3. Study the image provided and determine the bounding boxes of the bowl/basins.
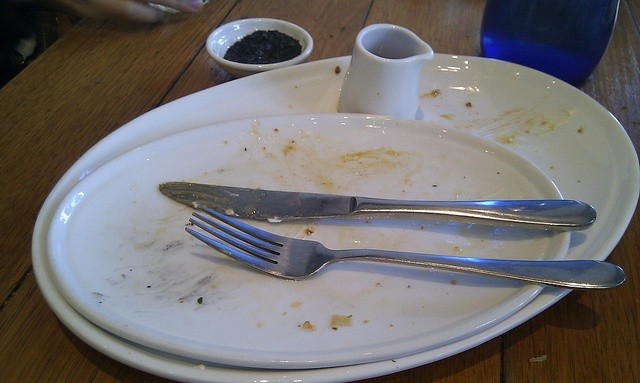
[207,17,313,76]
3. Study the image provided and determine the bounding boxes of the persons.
[1,1,205,86]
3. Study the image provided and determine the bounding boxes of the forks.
[183,205,625,293]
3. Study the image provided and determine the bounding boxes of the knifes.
[157,182,596,231]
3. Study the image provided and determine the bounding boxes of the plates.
[48,111,572,370]
[32,52,640,382]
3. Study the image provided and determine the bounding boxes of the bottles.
[337,22,433,119]
[481,1,618,88]
